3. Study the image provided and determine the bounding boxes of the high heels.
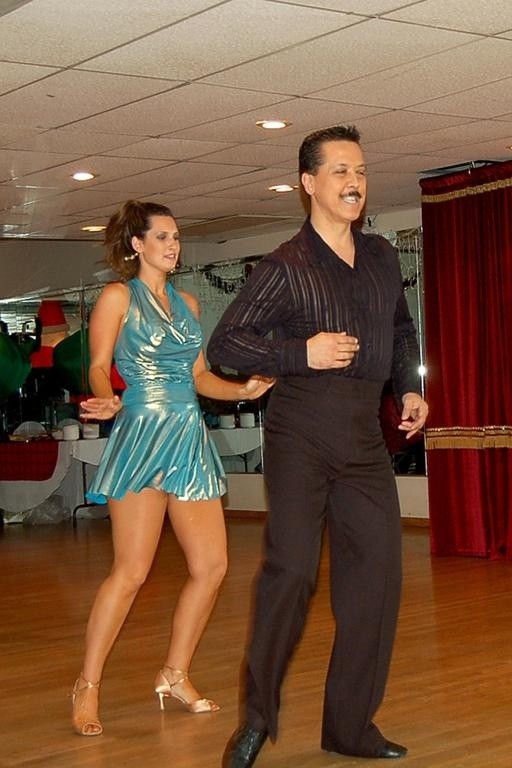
[152,666,222,715]
[71,671,102,737]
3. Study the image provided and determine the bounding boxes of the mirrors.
[0,227,429,476]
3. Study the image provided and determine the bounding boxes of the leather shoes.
[222,722,268,767]
[377,739,407,758]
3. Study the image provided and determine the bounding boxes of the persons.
[68,197,278,738]
[203,124,432,766]
[376,376,427,478]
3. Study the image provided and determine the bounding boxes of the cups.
[218,412,256,429]
[62,423,99,442]
